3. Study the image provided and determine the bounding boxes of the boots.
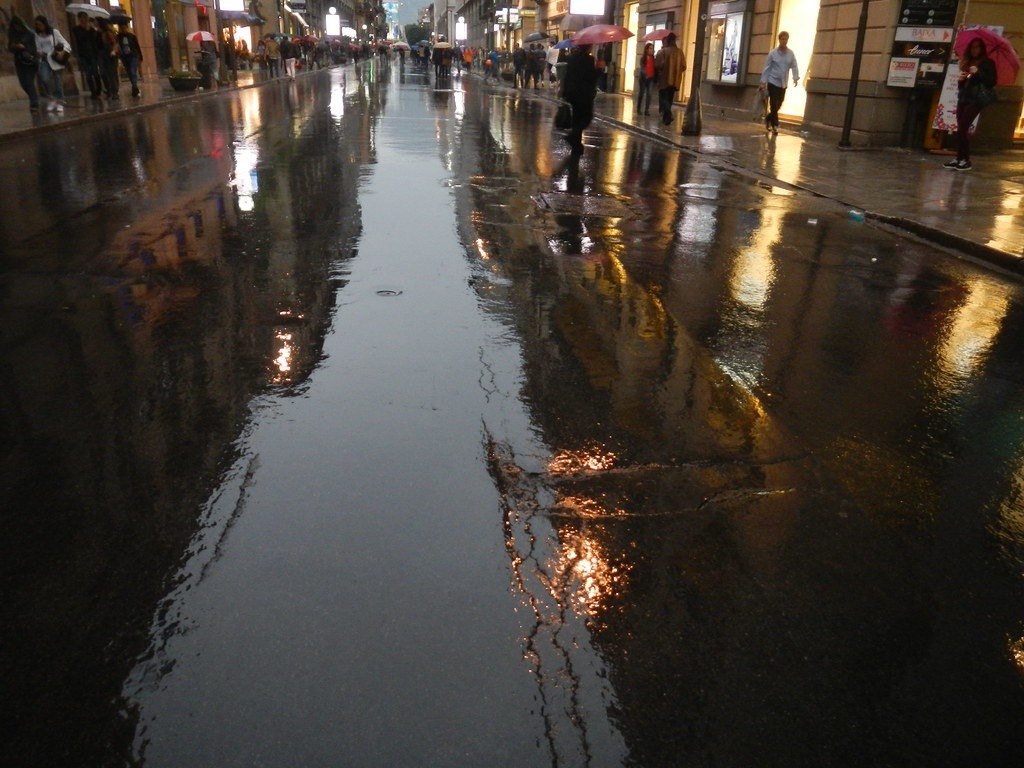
[637,91,644,114]
[645,95,651,115]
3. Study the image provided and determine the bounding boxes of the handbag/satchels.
[555,106,571,129]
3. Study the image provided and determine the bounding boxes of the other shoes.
[764,117,770,128]
[289,78,291,82]
[942,161,959,168]
[772,128,778,133]
[292,79,296,81]
[954,162,972,171]
[541,83,544,87]
[566,134,583,154]
[534,87,539,90]
[512,86,516,88]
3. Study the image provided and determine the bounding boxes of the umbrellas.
[106,13,133,24]
[570,24,636,44]
[65,3,110,20]
[263,31,452,50]
[554,39,577,49]
[642,28,681,40]
[524,31,550,43]
[954,28,1021,86]
[186,30,217,41]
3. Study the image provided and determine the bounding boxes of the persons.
[760,31,800,135]
[194,40,219,92]
[637,32,687,126]
[942,36,998,170]
[237,34,608,94]
[557,44,605,166]
[7,12,143,112]
